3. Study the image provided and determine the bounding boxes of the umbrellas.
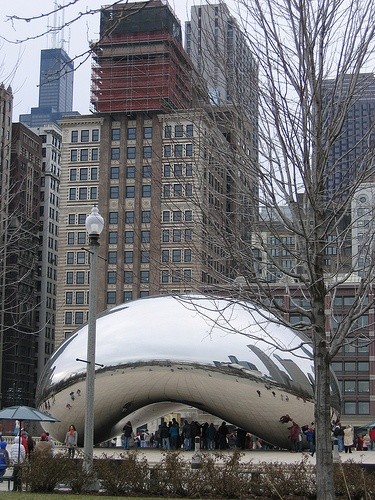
[0,405,62,464]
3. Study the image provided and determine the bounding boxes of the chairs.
[0,463,18,491]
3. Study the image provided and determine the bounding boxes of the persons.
[333,423,355,453]
[0,442,10,483]
[200,421,229,450]
[123,421,132,449]
[28,435,36,453]
[11,435,25,465]
[64,425,78,460]
[297,423,316,456]
[40,431,53,441]
[182,420,201,451]
[356,426,375,451]
[21,431,30,459]
[236,427,274,450]
[14,420,23,437]
[136,428,163,448]
[160,418,179,450]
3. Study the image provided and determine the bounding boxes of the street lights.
[77,201,106,493]
